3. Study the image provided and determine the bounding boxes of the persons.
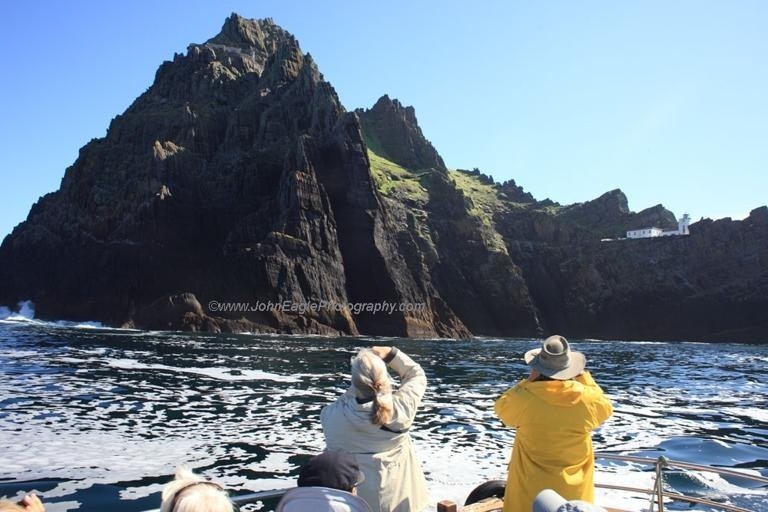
[273,450,375,512]
[319,347,428,511]
[533,489,609,511]
[0,495,45,512]
[491,336,613,511]
[160,470,234,512]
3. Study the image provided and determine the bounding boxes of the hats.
[296,450,366,490]
[523,333,588,383]
[530,488,607,512]
